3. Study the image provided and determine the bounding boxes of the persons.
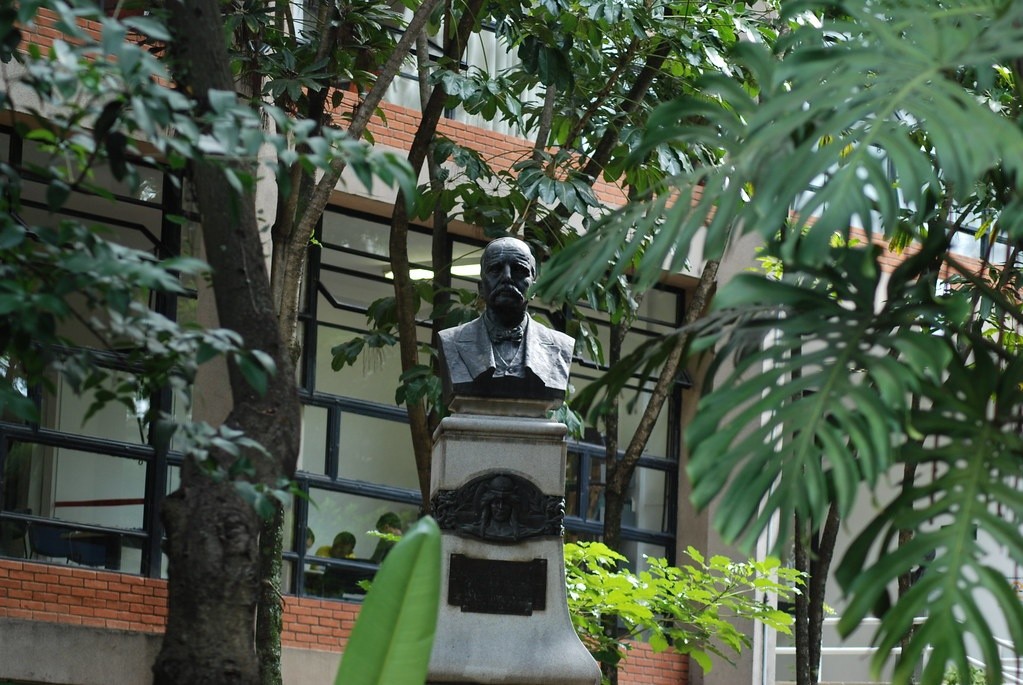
[376,511,402,560]
[310,532,356,570]
[306,527,315,549]
[438,236,575,400]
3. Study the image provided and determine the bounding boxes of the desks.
[62,530,167,570]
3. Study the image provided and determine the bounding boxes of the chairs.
[4,507,74,562]
